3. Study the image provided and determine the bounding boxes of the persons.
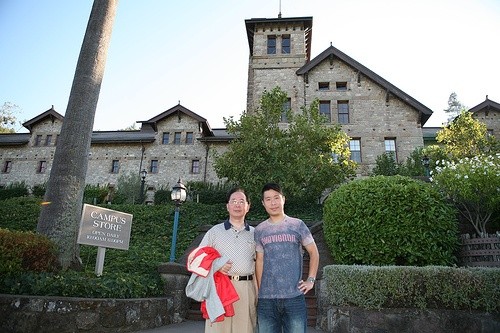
[253,185,320,333]
[196,188,258,333]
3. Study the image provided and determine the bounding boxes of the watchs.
[306,276,316,284]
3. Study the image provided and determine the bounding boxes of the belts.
[227,275,253,280]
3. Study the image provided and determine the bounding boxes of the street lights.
[139,169,147,204]
[168,177,188,261]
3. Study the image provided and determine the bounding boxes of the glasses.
[229,200,247,205]
